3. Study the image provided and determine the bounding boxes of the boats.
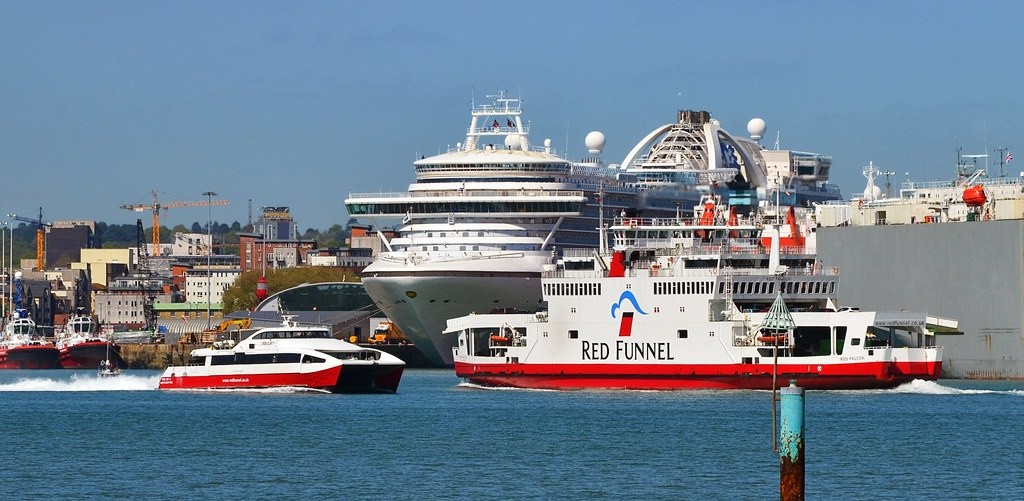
[159,326,409,399]
[0,311,63,370]
[439,182,966,393]
[96,361,123,379]
[55,307,127,369]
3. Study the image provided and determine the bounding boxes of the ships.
[345,87,992,369]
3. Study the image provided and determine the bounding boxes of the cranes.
[120,187,233,257]
[134,217,158,332]
[6,204,52,274]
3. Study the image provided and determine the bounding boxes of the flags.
[1005,152,1013,164]
